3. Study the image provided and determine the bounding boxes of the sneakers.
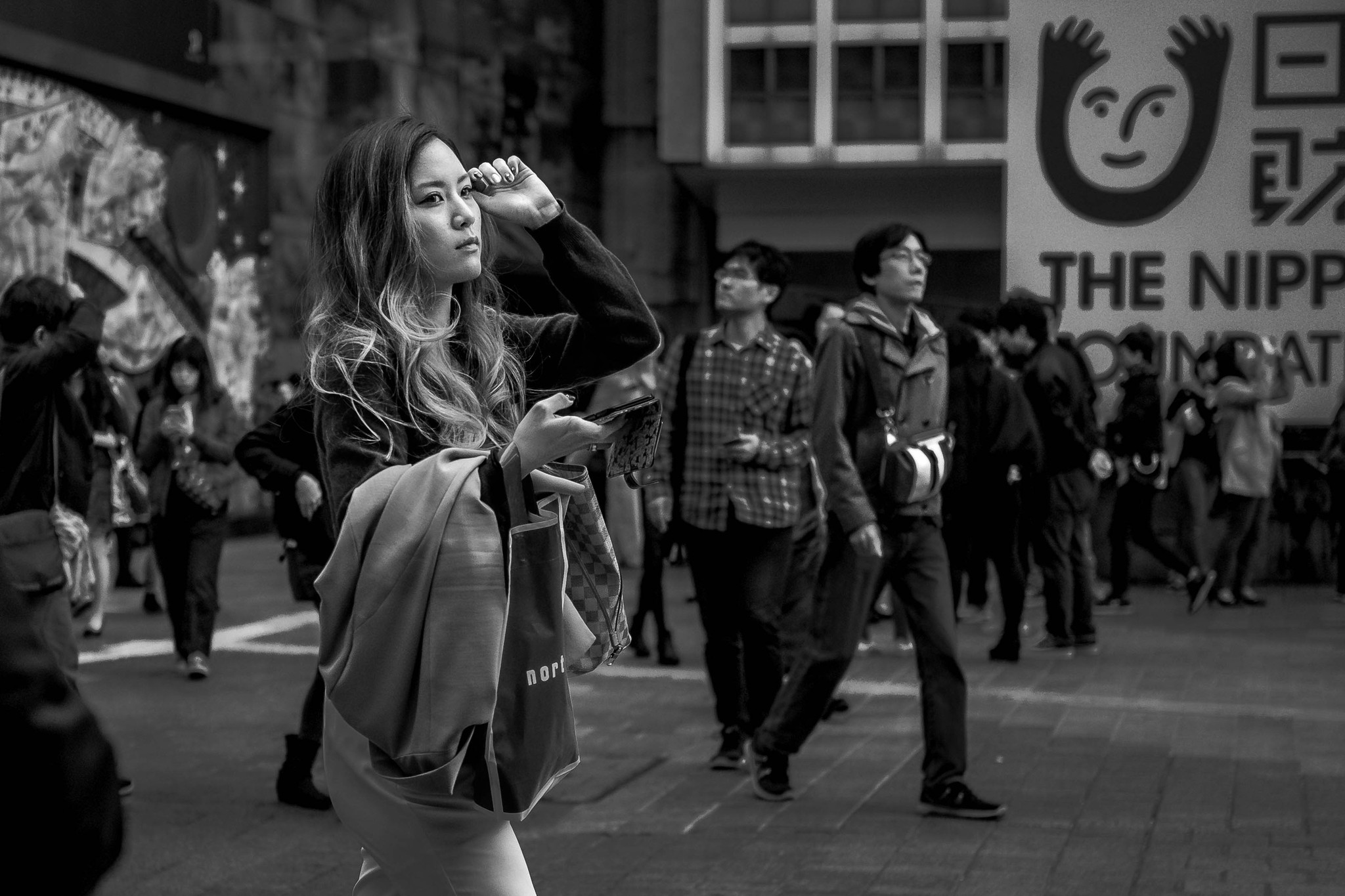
[1093,592,1133,611]
[916,781,1007,819]
[1186,568,1217,615]
[709,741,748,769]
[175,654,209,682]
[744,739,795,801]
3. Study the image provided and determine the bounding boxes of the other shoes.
[858,641,876,652]
[1207,585,1267,609]
[120,776,135,796]
[896,641,914,652]
[1031,630,1097,648]
[143,588,164,615]
[81,613,103,637]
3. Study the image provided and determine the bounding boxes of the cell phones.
[583,394,655,423]
[167,408,187,425]
[722,438,742,446]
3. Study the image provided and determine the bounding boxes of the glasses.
[712,266,761,283]
[880,246,934,266]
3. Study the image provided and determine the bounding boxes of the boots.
[629,613,649,658]
[114,534,146,589]
[657,628,676,665]
[989,615,1019,661]
[276,733,334,811]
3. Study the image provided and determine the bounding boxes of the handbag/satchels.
[879,428,956,505]
[495,401,634,671]
[659,487,690,567]
[290,551,335,604]
[0,508,80,673]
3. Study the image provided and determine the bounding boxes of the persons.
[573,284,1298,663]
[742,221,1009,819]
[0,273,166,896]
[307,120,666,896]
[135,331,239,680]
[651,242,817,770]
[232,392,336,814]
